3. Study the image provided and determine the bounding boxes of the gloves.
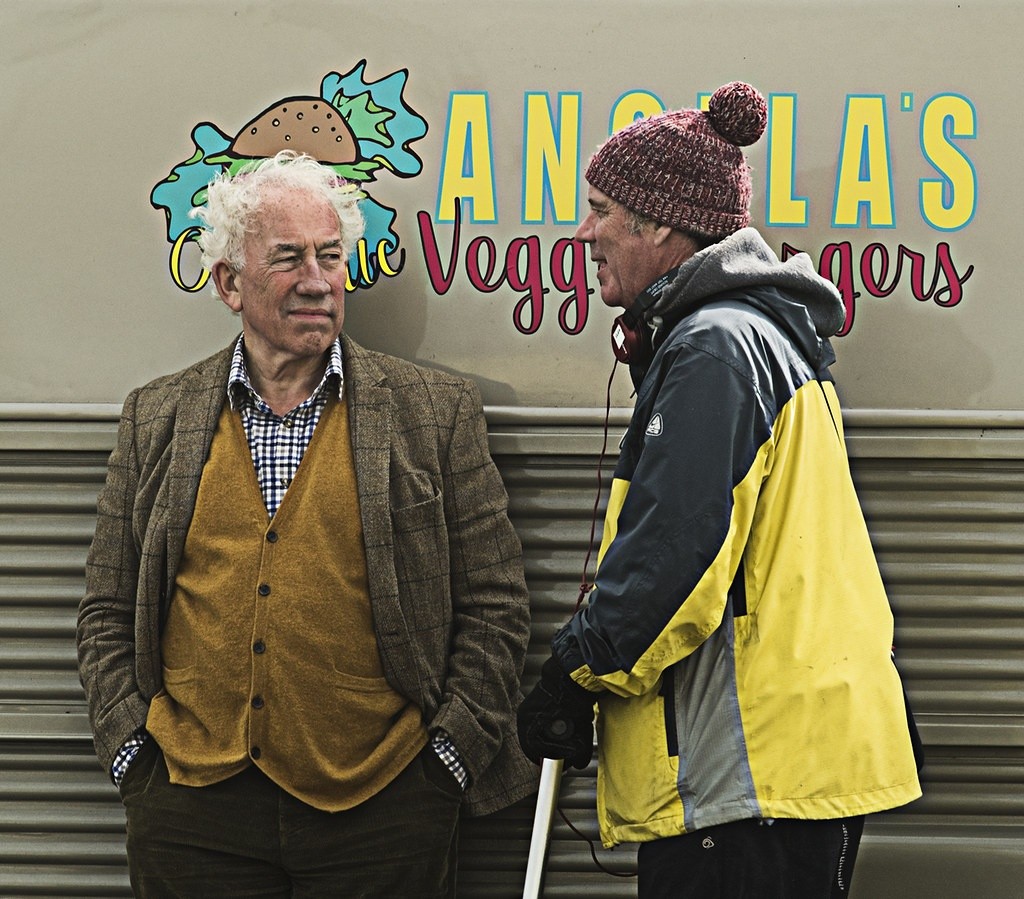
[515,658,595,770]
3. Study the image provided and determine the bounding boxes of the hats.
[587,80,766,239]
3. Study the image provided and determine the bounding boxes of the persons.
[75,149,533,899]
[517,80,925,899]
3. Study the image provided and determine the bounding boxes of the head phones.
[610,266,681,363]
